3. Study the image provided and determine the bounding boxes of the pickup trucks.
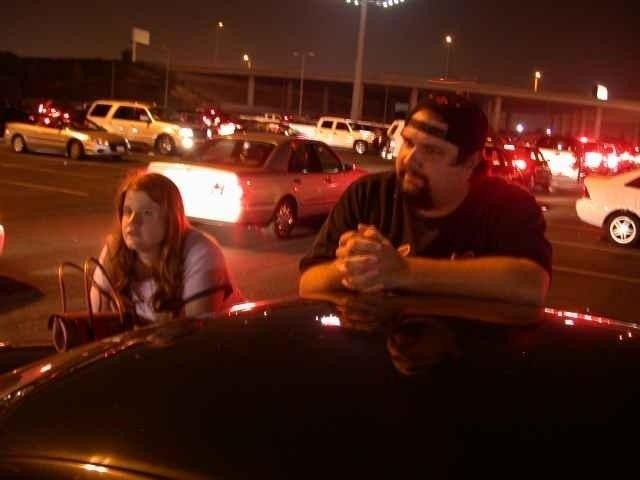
[288,116,377,155]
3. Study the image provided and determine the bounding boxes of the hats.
[404,89,489,150]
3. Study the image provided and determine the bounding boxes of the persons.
[89,173,248,329]
[307,291,548,377]
[298,94,552,309]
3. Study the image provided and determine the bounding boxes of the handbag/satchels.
[48,257,129,353]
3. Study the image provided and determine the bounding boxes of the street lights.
[344,0,407,122]
[444,35,452,80]
[291,50,313,116]
[213,20,225,63]
[534,71,541,94]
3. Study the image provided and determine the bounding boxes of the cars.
[240,111,285,132]
[380,120,406,159]
[0,286,639,477]
[579,137,640,175]
[148,133,377,242]
[531,134,583,182]
[483,144,553,194]
[576,167,640,249]
[4,98,131,162]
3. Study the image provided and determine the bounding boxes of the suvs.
[85,99,195,156]
